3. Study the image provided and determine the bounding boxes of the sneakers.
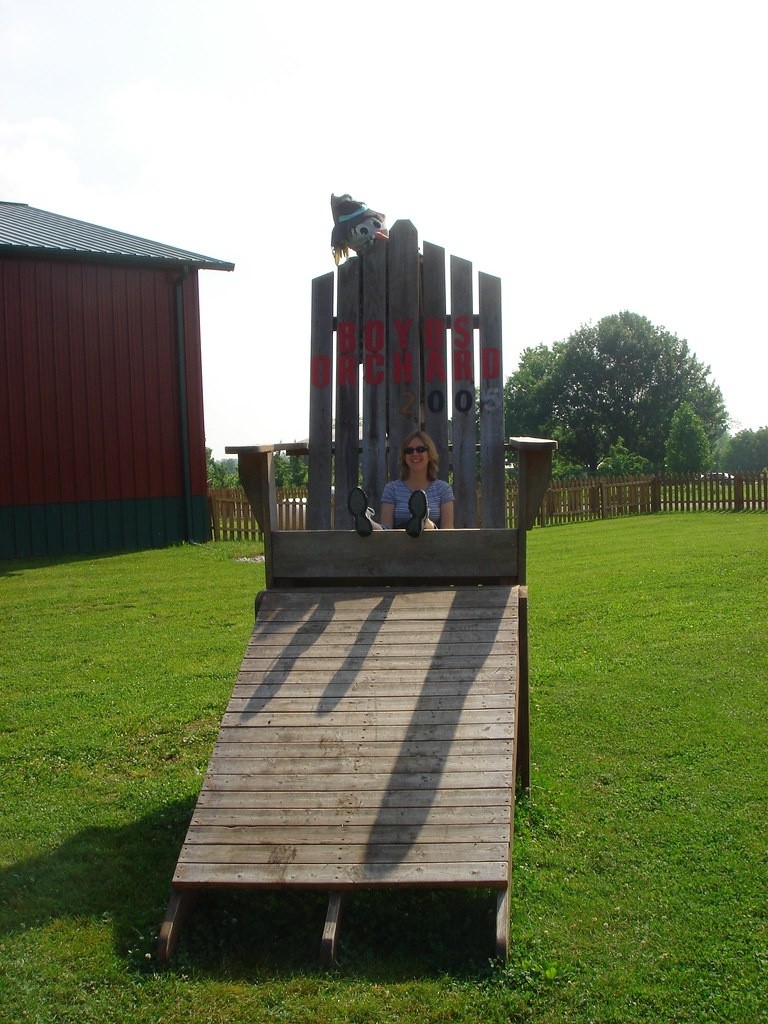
[406,490,429,538]
[348,486,372,537]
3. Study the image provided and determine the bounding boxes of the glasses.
[405,446,428,454]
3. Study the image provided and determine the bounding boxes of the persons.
[346,429,457,538]
